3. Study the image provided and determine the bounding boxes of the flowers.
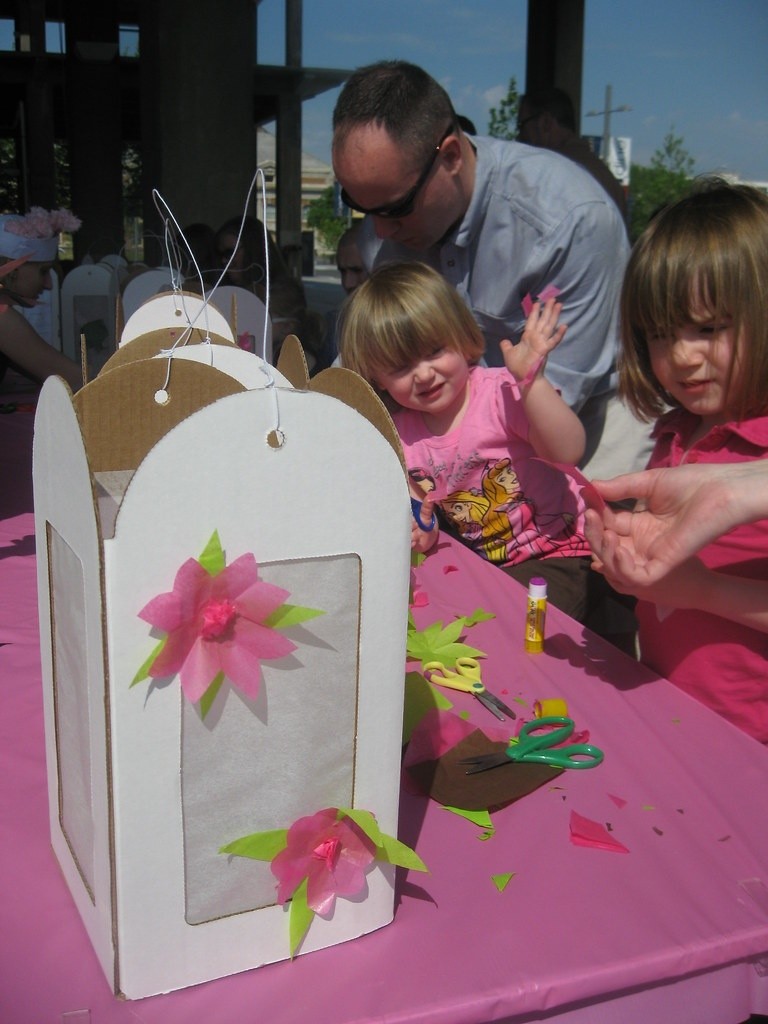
[127,528,326,723]
[218,806,428,962]
[6,206,81,239]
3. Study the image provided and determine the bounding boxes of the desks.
[0,512,768,1024]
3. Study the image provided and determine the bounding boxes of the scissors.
[457,716,604,775]
[423,657,516,721]
[411,496,435,531]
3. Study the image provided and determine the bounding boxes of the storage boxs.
[17,254,413,1001]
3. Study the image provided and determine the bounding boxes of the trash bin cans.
[302,229,314,276]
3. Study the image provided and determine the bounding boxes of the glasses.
[340,125,456,219]
[517,112,541,129]
[214,244,246,261]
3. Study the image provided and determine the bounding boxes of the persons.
[324,221,375,369]
[332,61,630,473]
[584,184,768,747]
[0,207,92,394]
[335,258,610,626]
[161,215,327,378]
[515,85,628,222]
